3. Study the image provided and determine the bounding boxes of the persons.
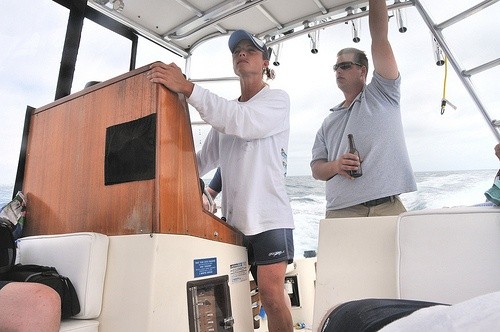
[147,28,295,332]
[310,0,418,219]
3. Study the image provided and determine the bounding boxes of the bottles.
[347,134,362,177]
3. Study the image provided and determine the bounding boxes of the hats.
[228,29,268,56]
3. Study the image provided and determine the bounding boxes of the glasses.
[333,61,362,71]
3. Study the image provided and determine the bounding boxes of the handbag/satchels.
[0,262,80,319]
[0,190,27,241]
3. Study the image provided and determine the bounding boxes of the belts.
[361,194,397,207]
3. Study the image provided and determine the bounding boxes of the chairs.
[397,207,500,304]
[14,232,109,332]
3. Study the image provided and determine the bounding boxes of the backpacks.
[0,222,17,266]
